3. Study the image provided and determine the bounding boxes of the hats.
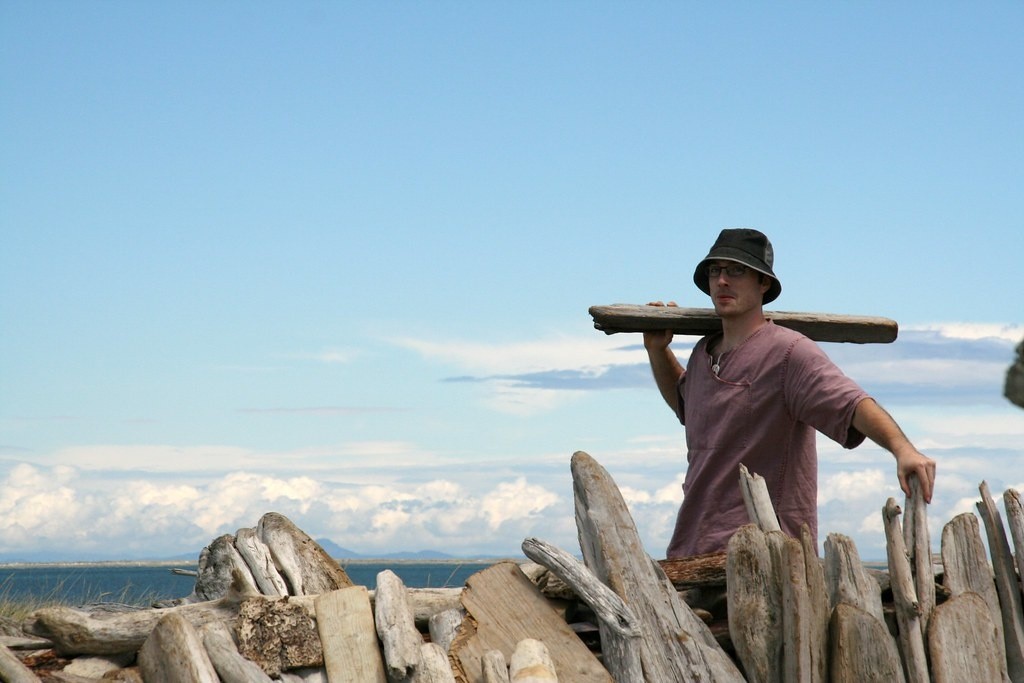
[693,228,782,305]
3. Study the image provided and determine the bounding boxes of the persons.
[643,229,935,559]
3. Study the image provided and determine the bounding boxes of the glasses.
[709,264,754,277]
